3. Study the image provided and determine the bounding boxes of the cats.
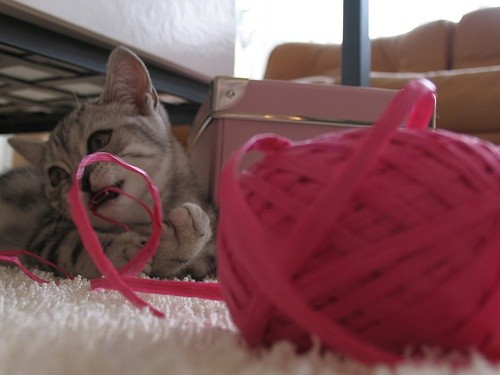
[0,44,217,282]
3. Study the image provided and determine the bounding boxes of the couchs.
[266,7,500,145]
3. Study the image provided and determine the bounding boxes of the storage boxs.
[184,74,438,212]
